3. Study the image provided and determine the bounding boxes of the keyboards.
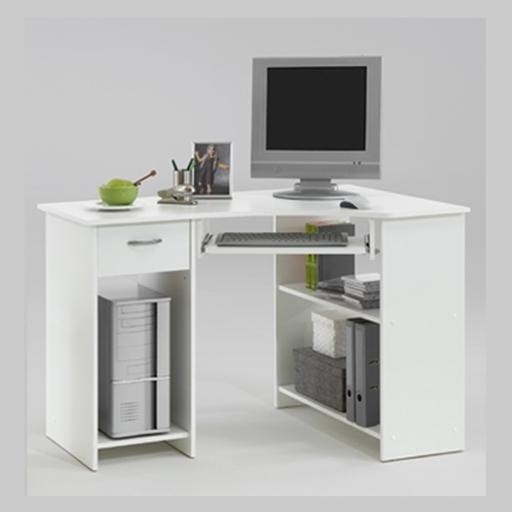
[216,231,349,247]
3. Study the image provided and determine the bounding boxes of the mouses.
[339,193,369,210]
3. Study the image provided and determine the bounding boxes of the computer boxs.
[98,280,172,439]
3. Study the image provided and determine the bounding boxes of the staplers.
[157,185,198,205]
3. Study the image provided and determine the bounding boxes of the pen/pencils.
[172,157,195,170]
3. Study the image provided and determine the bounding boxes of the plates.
[93,200,141,210]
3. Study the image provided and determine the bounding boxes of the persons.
[195,145,229,194]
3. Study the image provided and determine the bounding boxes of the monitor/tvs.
[250,56,383,200]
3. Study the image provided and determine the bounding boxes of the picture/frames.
[189,139,234,200]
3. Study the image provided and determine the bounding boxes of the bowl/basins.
[98,186,139,206]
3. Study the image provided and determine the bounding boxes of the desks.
[37,182,472,471]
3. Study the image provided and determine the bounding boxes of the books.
[316,272,380,310]
